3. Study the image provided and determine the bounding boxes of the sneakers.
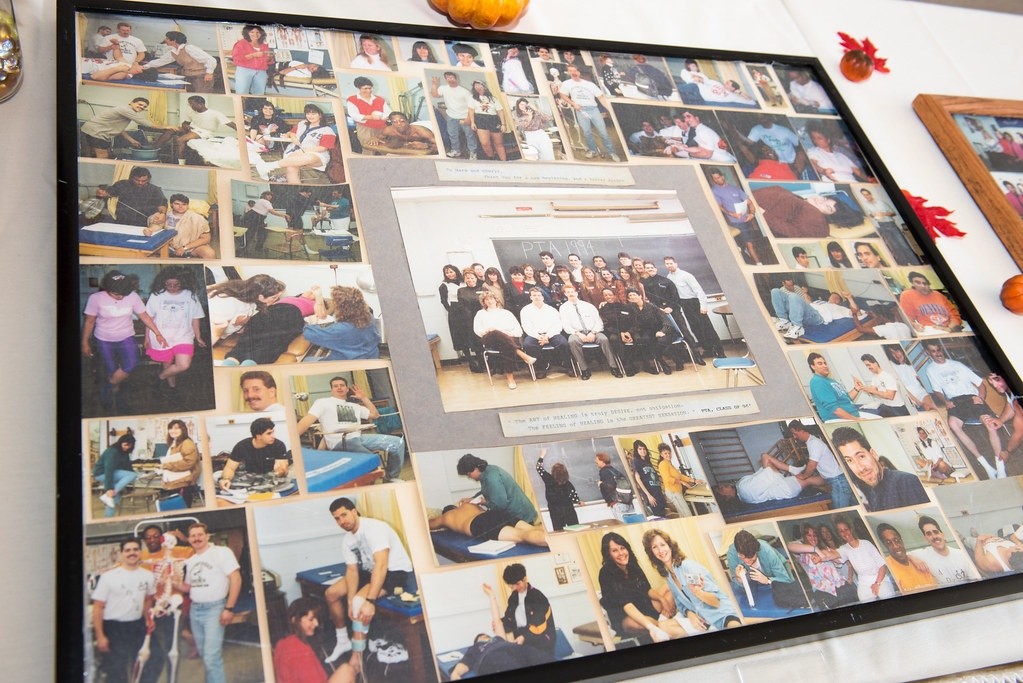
[776,318,792,331]
[368,639,409,664]
[784,325,805,338]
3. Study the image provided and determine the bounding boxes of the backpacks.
[608,468,633,504]
[635,65,659,97]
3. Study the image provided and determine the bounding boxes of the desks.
[78,222,178,259]
[712,304,735,345]
[296,562,428,683]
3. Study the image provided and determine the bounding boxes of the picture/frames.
[912,93,1023,272]
[54,0,1023,683]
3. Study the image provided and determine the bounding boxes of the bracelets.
[854,387,859,392]
[182,246,187,250]
[365,599,376,602]
[876,582,880,585]
[294,67,297,70]
[157,335,162,336]
[768,579,771,585]
[814,546,815,552]
[224,607,234,612]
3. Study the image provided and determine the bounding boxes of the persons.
[82,23,1023,683]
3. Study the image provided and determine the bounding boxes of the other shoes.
[537,362,550,379]
[525,356,537,364]
[447,149,461,157]
[696,355,707,366]
[99,493,115,508]
[586,150,598,158]
[582,368,590,380]
[718,350,725,359]
[611,152,620,162]
[508,379,516,389]
[470,151,477,160]
[676,359,683,371]
[661,361,671,376]
[701,351,713,358]
[610,366,623,378]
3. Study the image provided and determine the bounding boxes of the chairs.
[712,340,766,389]
[267,230,358,263]
[481,312,703,384]
